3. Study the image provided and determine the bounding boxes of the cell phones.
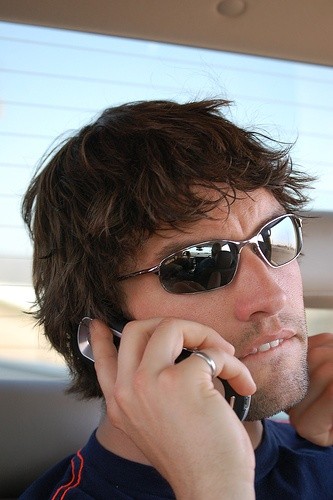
[75,316,251,422]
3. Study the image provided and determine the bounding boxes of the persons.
[19,98,332,500]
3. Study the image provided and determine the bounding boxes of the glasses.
[115,213,304,295]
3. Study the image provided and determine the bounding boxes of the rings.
[193,350,215,381]
